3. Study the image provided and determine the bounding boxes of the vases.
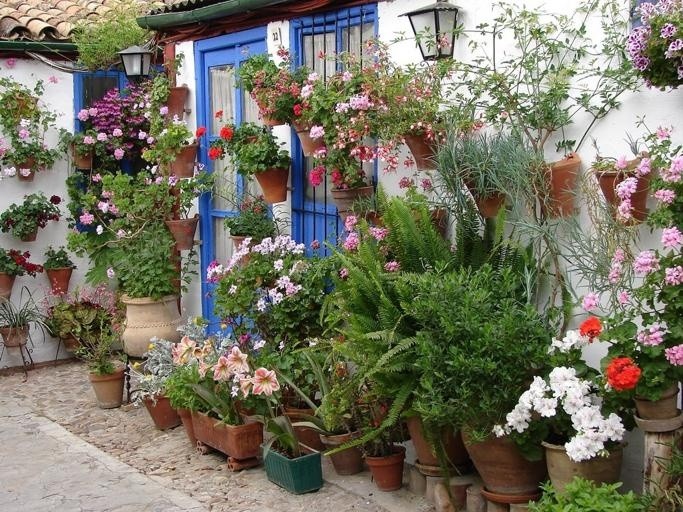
[366,446,406,490]
[460,425,546,495]
[541,439,622,499]
[264,440,322,495]
[320,434,366,476]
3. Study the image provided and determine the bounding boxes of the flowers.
[0,0,683,460]
